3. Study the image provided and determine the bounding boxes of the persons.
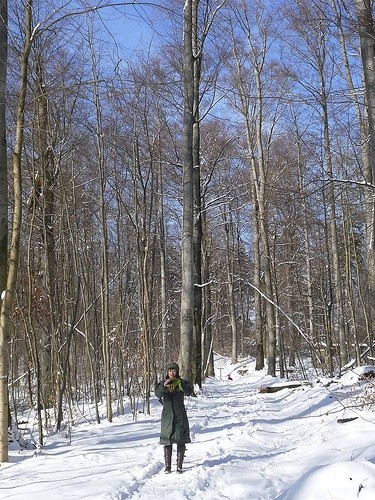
[155,362,194,474]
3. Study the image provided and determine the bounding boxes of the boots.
[163,445,171,474]
[176,445,186,473]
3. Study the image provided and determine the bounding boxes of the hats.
[166,363,179,375]
